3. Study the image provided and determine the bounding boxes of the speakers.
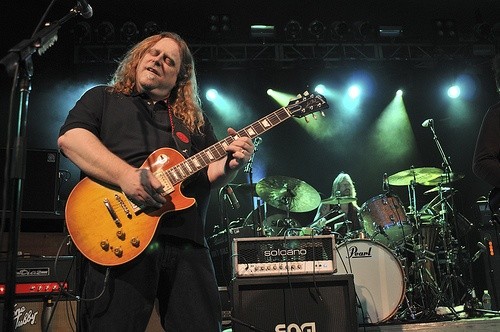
[0,147,60,213]
[0,295,79,332]
[228,274,357,332]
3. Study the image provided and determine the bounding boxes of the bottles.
[482,290,491,309]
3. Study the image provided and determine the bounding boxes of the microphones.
[76,0,93,19]
[227,184,241,209]
[421,118,433,127]
[245,137,262,173]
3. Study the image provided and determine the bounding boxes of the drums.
[412,219,442,297]
[335,238,407,325]
[359,192,414,246]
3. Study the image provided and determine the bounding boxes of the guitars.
[64,89,331,268]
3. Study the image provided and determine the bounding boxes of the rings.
[241,149,245,153]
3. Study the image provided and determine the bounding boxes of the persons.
[313,174,365,238]
[56,32,255,332]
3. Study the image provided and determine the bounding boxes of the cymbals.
[422,186,455,199]
[385,166,444,185]
[262,214,302,237]
[255,175,322,213]
[418,172,465,186]
[320,195,358,206]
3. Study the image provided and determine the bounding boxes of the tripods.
[384,128,477,322]
